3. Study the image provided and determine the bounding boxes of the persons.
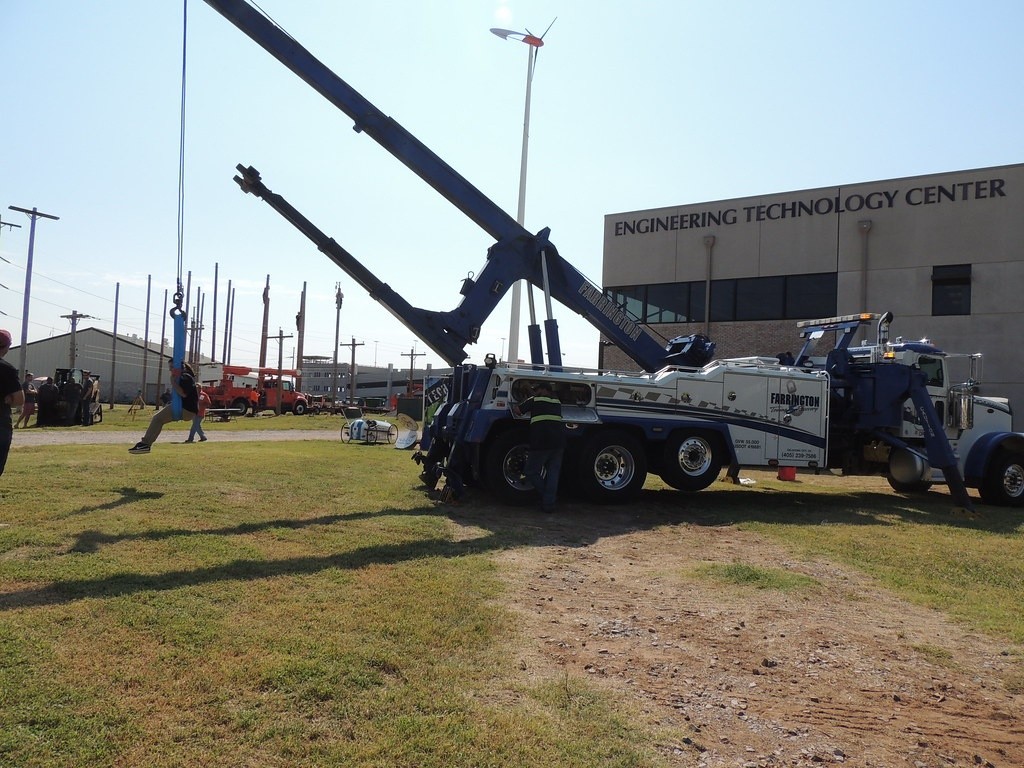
[160,388,171,408]
[36,377,59,427]
[257,389,266,410]
[63,372,94,427]
[185,383,211,442]
[251,387,258,414]
[128,358,199,454]
[514,381,566,514]
[14,373,38,428]
[0,330,26,529]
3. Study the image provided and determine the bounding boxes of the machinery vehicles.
[199,365,308,416]
[37,368,102,426]
[203,0,1024,509]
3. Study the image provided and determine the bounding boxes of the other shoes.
[185,440,193,443]
[198,437,208,442]
[128,442,151,453]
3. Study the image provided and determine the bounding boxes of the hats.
[195,383,202,388]
[0,329,12,346]
